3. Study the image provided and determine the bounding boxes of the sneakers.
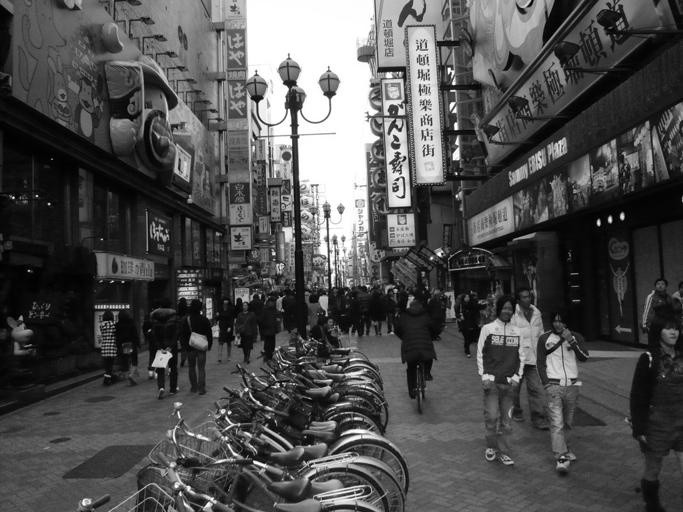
[532,416,550,430]
[425,374,433,380]
[411,392,416,399]
[566,450,576,461]
[466,352,471,358]
[512,406,523,421]
[191,388,197,393]
[170,386,179,395]
[199,390,206,394]
[485,447,496,461]
[157,387,165,399]
[148,371,154,379]
[555,455,570,473]
[498,453,514,466]
[154,372,159,379]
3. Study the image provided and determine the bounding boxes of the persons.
[509,287,548,429]
[536,308,590,474]
[94,289,297,398]
[641,276,671,333]
[671,282,682,306]
[304,286,488,400]
[475,294,526,465]
[629,310,681,512]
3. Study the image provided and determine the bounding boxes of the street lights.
[308,197,348,295]
[247,53,340,341]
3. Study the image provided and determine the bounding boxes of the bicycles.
[411,356,430,414]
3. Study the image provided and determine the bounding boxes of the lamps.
[470,9,682,146]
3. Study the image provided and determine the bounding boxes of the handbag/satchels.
[238,324,246,333]
[189,331,209,351]
[234,336,241,345]
[457,312,464,322]
[151,350,174,369]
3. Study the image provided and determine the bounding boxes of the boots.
[111,375,121,383]
[104,374,112,385]
[641,478,668,512]
[123,371,133,386]
[129,366,138,384]
